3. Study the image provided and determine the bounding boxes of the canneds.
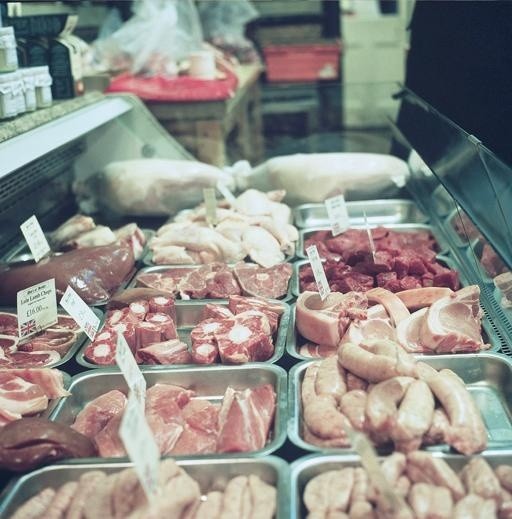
[0,25,54,119]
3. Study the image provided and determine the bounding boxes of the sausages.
[7,459,278,519]
[303,453,511,519]
[302,337,488,455]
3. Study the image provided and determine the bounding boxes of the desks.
[105,58,266,172]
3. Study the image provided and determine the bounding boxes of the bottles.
[0,26,54,121]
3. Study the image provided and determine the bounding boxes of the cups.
[187,52,218,80]
